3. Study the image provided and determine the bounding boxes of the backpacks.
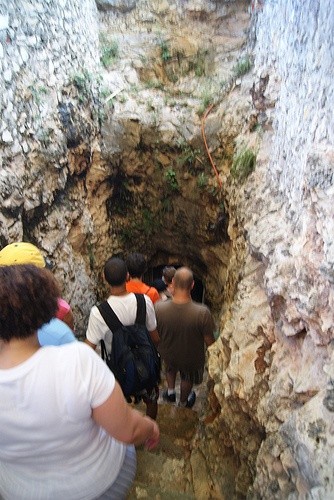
[95,293,162,399]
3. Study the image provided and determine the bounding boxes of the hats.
[0,242,46,269]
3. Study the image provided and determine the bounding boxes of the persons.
[124,256,161,307]
[0,241,79,346]
[53,298,76,333]
[85,257,162,422]
[153,280,169,302]
[160,266,178,301]
[0,262,161,500]
[153,266,217,410]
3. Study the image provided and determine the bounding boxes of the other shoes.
[178,391,196,409]
[163,388,177,403]
